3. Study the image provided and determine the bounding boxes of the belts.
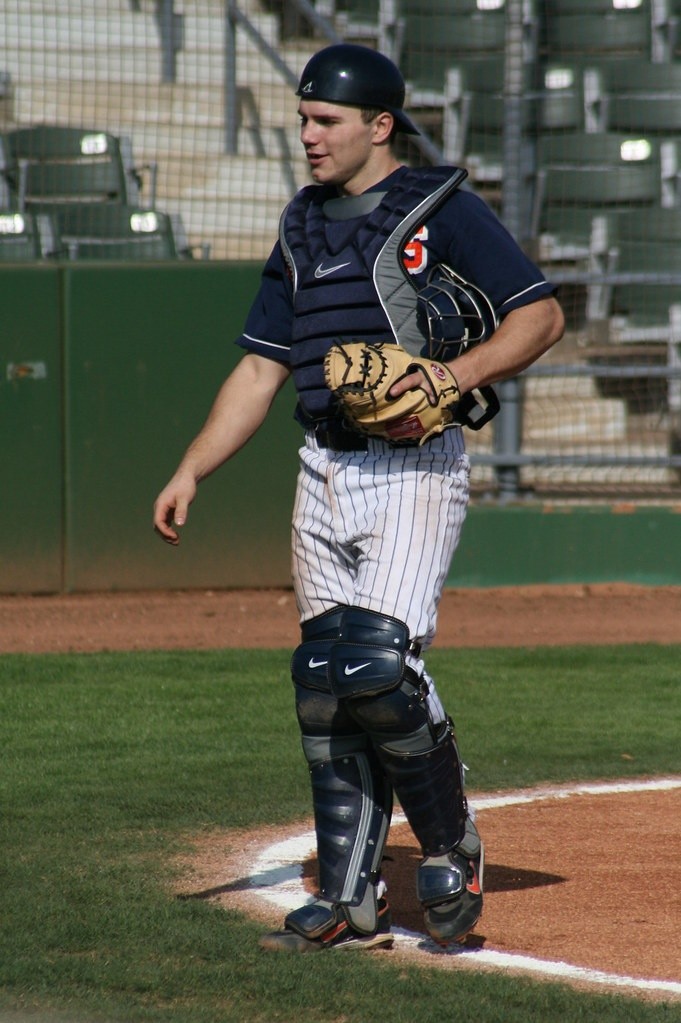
[315,429,444,453]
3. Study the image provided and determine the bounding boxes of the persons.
[153,48,566,956]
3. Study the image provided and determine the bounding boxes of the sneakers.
[257,896,394,953]
[417,816,484,949]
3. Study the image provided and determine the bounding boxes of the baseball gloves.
[323,336,465,451]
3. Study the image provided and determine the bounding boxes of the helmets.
[295,44,422,137]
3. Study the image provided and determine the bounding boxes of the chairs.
[0,0,681,411]
